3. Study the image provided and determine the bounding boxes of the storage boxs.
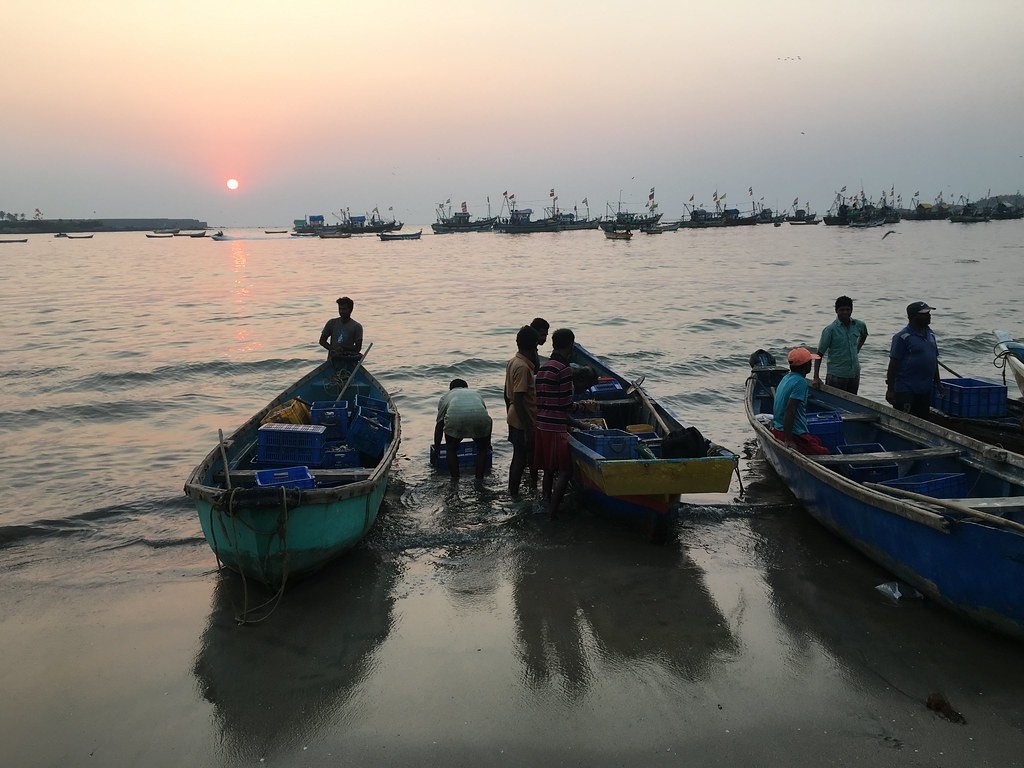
[429,440,493,471]
[250,392,394,487]
[808,377,1009,500]
[570,378,663,460]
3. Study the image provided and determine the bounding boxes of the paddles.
[333,342,373,408]
[627,375,670,435]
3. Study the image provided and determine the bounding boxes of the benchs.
[810,448,967,465]
[842,411,876,422]
[229,469,373,480]
[572,398,640,408]
[940,495,1024,512]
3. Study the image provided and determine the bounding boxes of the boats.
[604,230,632,239]
[183,349,401,582]
[265,230,287,233]
[0,240,28,243]
[153,230,180,233]
[376,230,423,239]
[567,340,740,512]
[54,232,67,237]
[319,234,351,237]
[291,210,405,233]
[68,235,95,238]
[174,232,206,236]
[291,233,317,236]
[431,192,1024,234]
[992,329,1024,396]
[146,234,173,238]
[929,397,1024,456]
[746,350,1023,634]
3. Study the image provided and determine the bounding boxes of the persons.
[319,297,363,359]
[433,379,493,480]
[885,302,945,420]
[504,318,585,506]
[812,296,868,396]
[772,348,829,456]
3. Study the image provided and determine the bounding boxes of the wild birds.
[882,230,903,240]
[777,56,802,60]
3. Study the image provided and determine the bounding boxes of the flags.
[793,197,798,205]
[503,191,507,195]
[713,192,726,201]
[461,202,467,212]
[749,187,752,196]
[582,198,587,203]
[509,194,514,199]
[861,188,900,202]
[550,189,554,196]
[649,187,654,200]
[915,191,919,196]
[841,186,846,192]
[373,208,378,212]
[689,196,694,201]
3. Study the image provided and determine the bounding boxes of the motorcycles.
[211,233,225,240]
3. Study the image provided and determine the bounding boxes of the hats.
[907,301,936,316]
[788,348,822,366]
[337,298,353,307]
